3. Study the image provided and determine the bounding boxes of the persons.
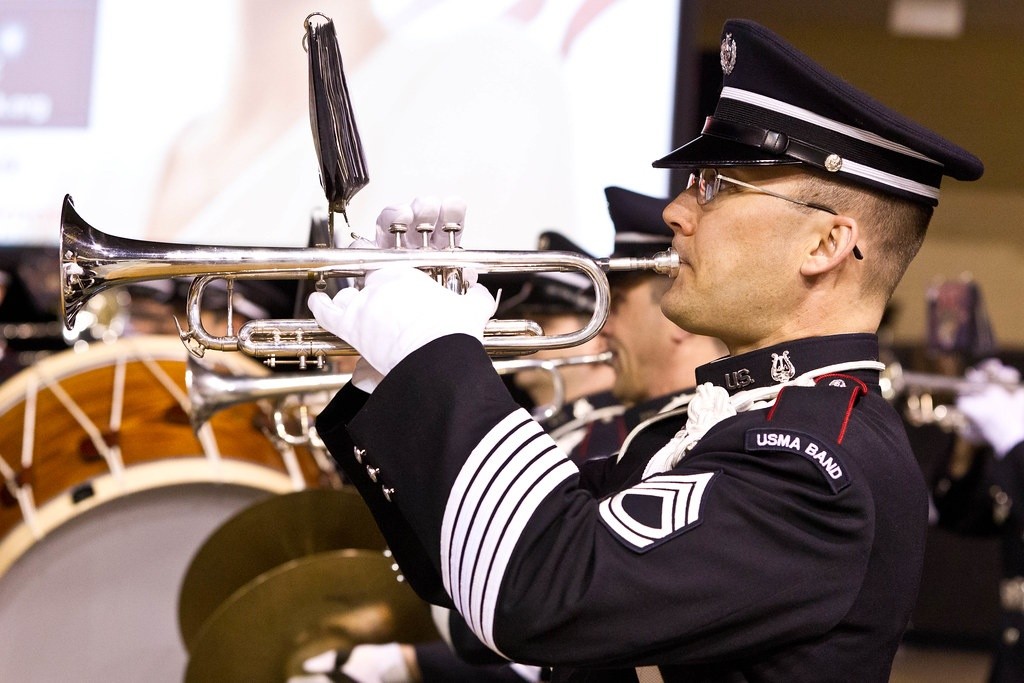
[309,18,984,683]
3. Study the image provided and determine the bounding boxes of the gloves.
[308,265,497,395]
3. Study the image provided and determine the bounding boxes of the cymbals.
[185,552,456,681]
[178,487,390,656]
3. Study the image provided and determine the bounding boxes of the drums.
[0,333,331,683]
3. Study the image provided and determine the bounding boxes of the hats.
[604,186,675,281]
[522,233,603,316]
[653,19,984,208]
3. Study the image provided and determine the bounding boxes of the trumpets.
[184,342,622,451]
[60,195,677,427]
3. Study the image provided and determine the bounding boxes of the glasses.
[686,167,864,261]
